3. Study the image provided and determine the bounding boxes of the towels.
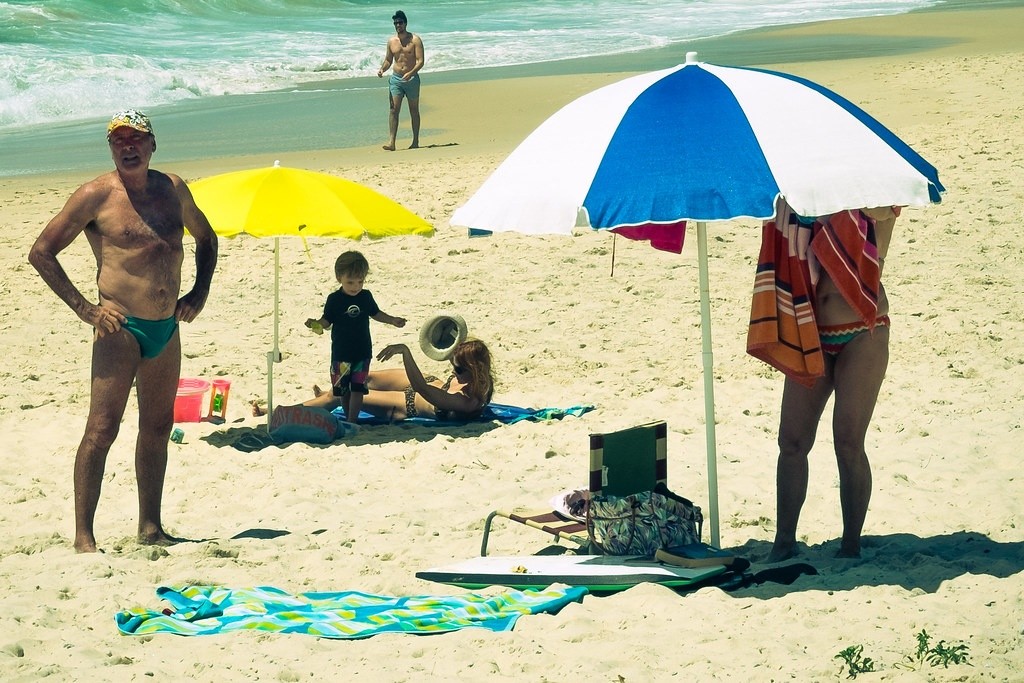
[331,397,596,428]
[746,195,880,391]
[113,577,592,641]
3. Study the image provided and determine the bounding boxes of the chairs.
[482,419,703,564]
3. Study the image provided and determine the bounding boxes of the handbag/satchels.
[585,482,700,556]
[269,405,346,445]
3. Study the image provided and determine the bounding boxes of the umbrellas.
[174,158,437,434]
[451,53,948,552]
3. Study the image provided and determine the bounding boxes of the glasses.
[570,499,585,515]
[394,21,403,25]
[452,365,468,374]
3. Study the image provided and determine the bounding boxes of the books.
[653,539,735,568]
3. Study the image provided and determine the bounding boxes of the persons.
[252,249,495,427]
[759,205,902,563]
[26,108,220,557]
[378,10,425,150]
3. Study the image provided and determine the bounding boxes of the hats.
[392,11,407,24]
[419,311,467,361]
[106,108,155,141]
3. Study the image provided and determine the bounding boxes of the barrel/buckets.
[173,377,209,423]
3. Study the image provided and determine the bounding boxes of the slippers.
[230,429,283,453]
[718,570,754,592]
[755,563,819,584]
